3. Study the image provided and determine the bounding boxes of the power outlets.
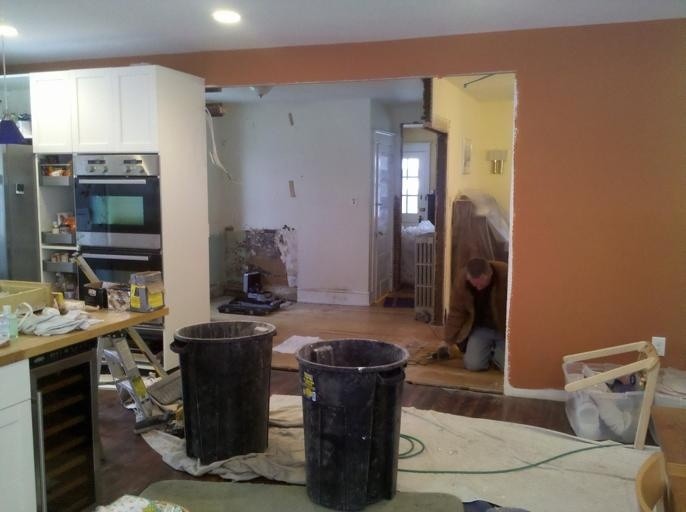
[653,336,667,358]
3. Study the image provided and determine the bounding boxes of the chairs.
[635,451,669,511]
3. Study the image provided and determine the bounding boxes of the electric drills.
[426,345,449,362]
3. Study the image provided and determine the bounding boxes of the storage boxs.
[562,360,644,445]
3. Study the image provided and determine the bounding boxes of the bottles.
[52,221,71,235]
[0,304,19,340]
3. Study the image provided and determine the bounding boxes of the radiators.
[414,233,435,323]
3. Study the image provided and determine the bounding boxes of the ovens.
[74,177,163,327]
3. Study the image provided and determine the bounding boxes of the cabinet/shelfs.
[452,201,508,302]
[30,63,206,374]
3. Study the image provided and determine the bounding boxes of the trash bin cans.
[295,338,410,512]
[170,321,277,465]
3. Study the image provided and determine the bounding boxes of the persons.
[437,255,508,374]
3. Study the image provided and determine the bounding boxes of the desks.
[650,405,686,512]
[2,307,170,510]
